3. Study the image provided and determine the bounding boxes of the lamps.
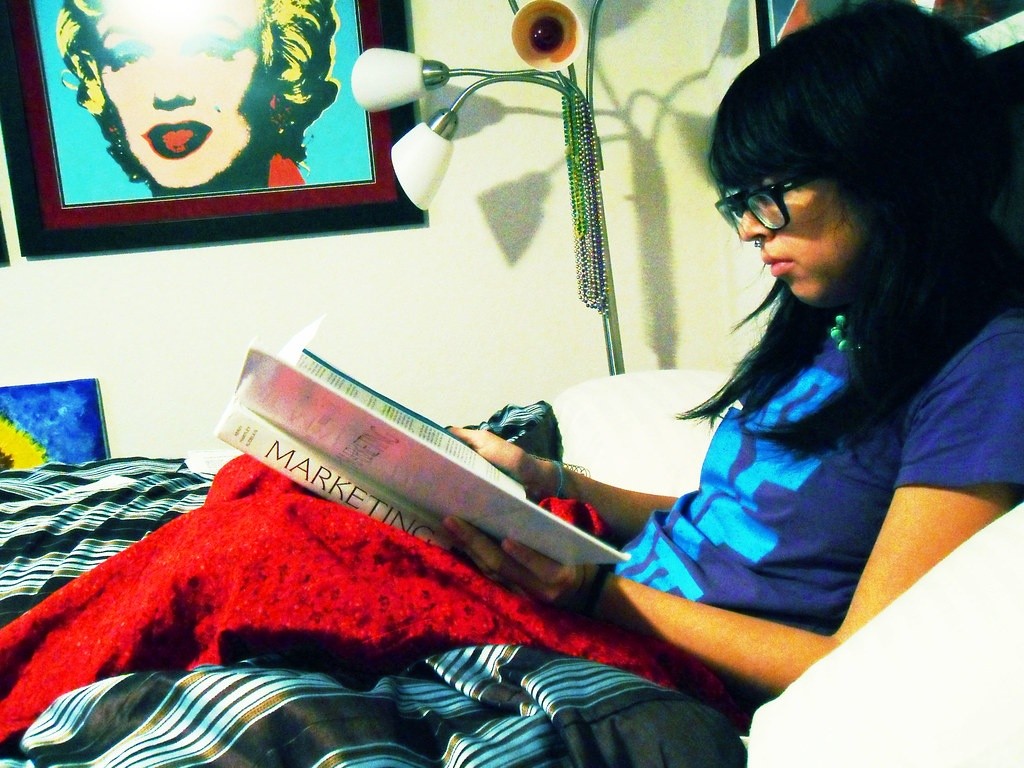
[350,0,624,376]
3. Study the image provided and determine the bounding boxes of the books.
[216,346,630,575]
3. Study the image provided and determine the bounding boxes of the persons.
[442,0,1024,719]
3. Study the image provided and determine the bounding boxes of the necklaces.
[831,314,867,351]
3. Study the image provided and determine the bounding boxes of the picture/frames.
[1,0,425,259]
[752,1,1023,74]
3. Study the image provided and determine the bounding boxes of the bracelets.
[554,460,563,498]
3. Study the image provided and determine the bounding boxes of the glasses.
[715,172,822,230]
